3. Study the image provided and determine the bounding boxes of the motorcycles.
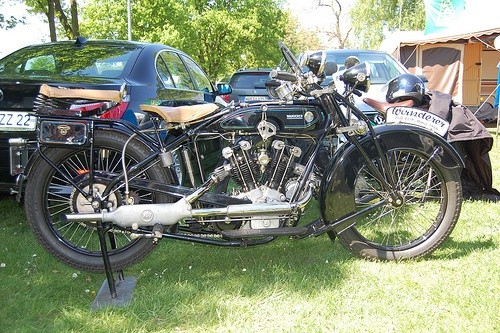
[10,42,465,301]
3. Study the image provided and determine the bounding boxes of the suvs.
[218,67,284,107]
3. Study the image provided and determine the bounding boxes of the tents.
[378,0,500,116]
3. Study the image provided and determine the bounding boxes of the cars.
[1,40,233,196]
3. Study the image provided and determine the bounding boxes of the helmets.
[386,73,425,107]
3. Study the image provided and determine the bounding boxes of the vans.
[298,48,434,127]
